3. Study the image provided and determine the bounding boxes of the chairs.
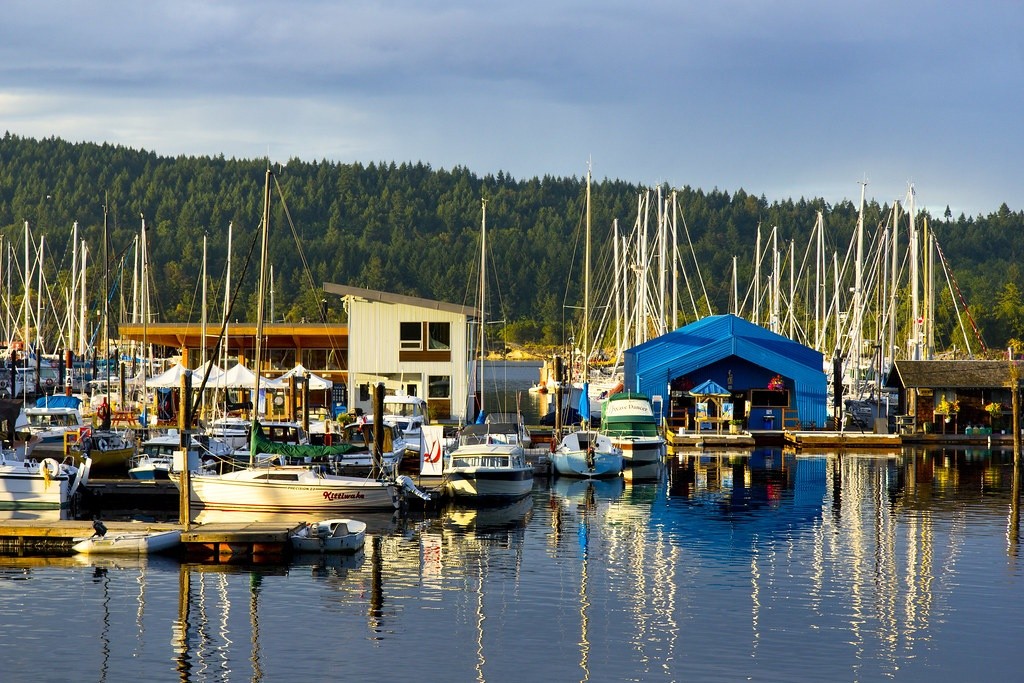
[332,523,349,537]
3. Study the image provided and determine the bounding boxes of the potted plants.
[337,412,353,426]
[727,419,744,434]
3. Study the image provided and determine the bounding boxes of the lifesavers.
[39,458,58,478]
[46,378,54,386]
[98,438,108,452]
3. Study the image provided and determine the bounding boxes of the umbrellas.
[146,360,332,419]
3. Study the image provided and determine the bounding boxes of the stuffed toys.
[768,376,784,390]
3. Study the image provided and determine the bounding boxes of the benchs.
[111,418,139,427]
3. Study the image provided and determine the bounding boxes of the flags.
[420,425,443,475]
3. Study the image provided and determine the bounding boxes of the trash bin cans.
[763,416,775,430]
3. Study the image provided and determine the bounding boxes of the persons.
[675,376,698,427]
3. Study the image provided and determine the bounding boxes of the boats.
[485,412,532,447]
[17,407,136,466]
[231,422,313,465]
[600,392,665,462]
[443,494,535,536]
[0,441,92,507]
[290,519,366,553]
[72,519,182,552]
[444,444,534,497]
[383,395,428,451]
[127,438,216,479]
[328,420,407,474]
[605,462,662,527]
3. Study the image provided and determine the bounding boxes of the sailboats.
[553,477,627,683]
[0,219,151,399]
[548,170,623,478]
[205,222,252,449]
[168,169,408,512]
[187,509,395,682]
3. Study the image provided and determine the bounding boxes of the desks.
[112,412,136,426]
